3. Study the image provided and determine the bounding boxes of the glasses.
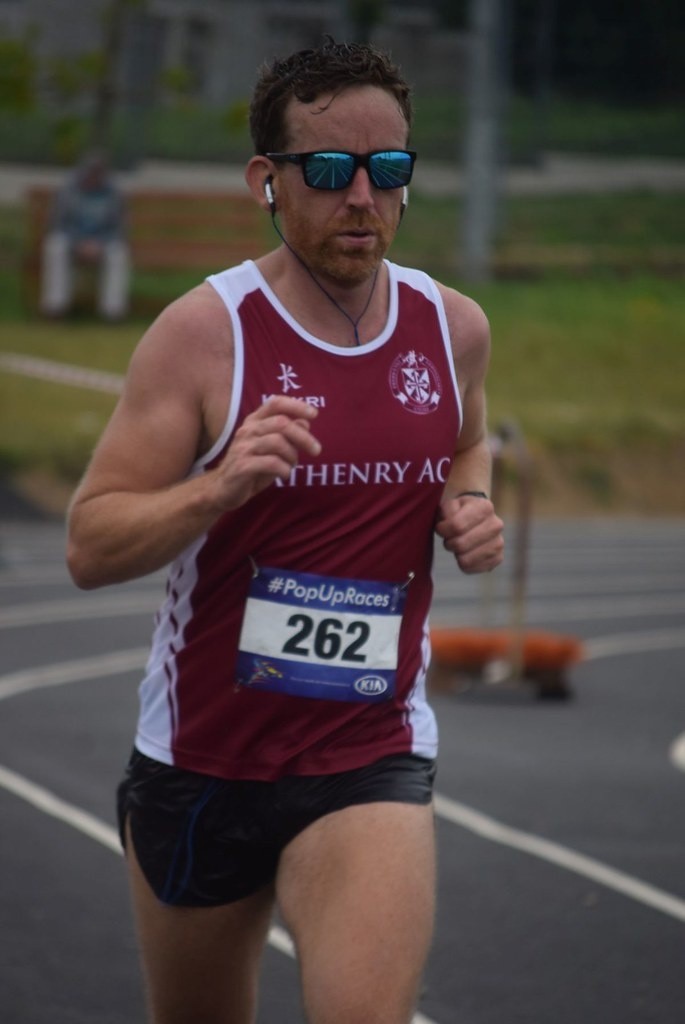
[265,150,416,192]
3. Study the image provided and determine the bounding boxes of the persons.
[42,150,130,318]
[67,43,507,1024]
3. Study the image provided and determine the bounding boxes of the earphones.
[400,187,408,218]
[264,174,277,216]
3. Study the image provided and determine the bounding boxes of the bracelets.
[457,491,487,497]
